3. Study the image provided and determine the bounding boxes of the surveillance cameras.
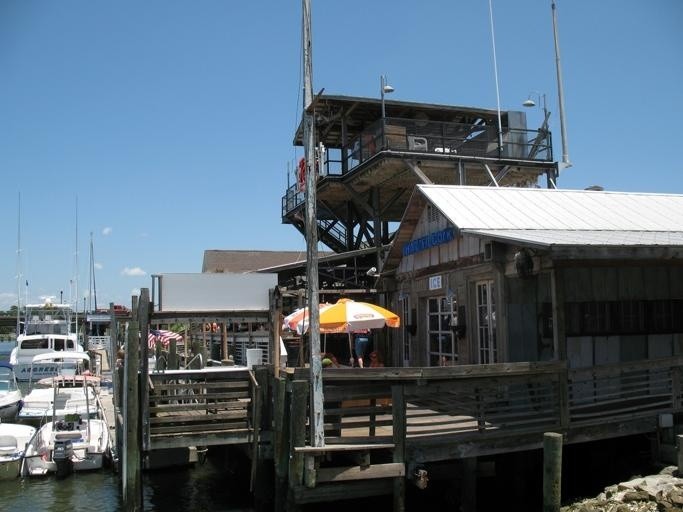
[366,267,377,276]
[512,251,534,281]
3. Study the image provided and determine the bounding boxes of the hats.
[322,358,333,368]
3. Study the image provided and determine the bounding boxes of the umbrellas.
[295,297,400,359]
[281,301,334,354]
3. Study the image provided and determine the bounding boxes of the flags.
[145,328,184,350]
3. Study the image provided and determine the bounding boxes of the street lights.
[522,89,551,160]
[379,74,393,150]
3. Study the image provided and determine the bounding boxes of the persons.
[324,332,386,368]
[199,322,269,332]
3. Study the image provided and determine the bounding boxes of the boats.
[0,189,110,481]
